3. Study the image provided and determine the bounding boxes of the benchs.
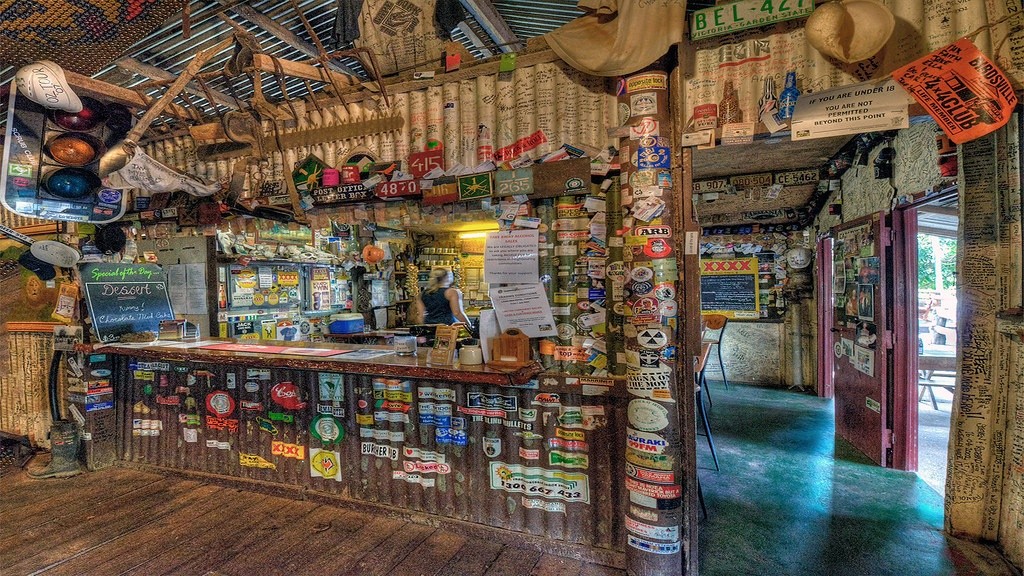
[920,381,955,394]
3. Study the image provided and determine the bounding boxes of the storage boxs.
[329,313,364,334]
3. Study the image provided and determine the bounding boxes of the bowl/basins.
[41,95,107,201]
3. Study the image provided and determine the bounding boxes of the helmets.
[16,59,83,112]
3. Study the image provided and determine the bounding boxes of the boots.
[26,421,81,478]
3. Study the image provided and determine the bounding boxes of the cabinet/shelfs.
[419,253,457,267]
[393,270,429,303]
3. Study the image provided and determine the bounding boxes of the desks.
[324,328,409,344]
[918,351,956,410]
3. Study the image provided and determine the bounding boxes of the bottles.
[459,338,483,365]
[778,66,800,120]
[717,80,740,126]
[393,331,417,356]
[757,76,778,122]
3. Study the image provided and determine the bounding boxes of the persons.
[416,267,471,326]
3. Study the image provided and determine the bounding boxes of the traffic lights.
[3,77,140,225]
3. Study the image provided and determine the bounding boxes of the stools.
[693,315,728,518]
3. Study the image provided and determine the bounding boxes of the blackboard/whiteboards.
[74,261,175,342]
[701,275,756,311]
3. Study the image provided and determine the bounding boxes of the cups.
[363,324,370,333]
